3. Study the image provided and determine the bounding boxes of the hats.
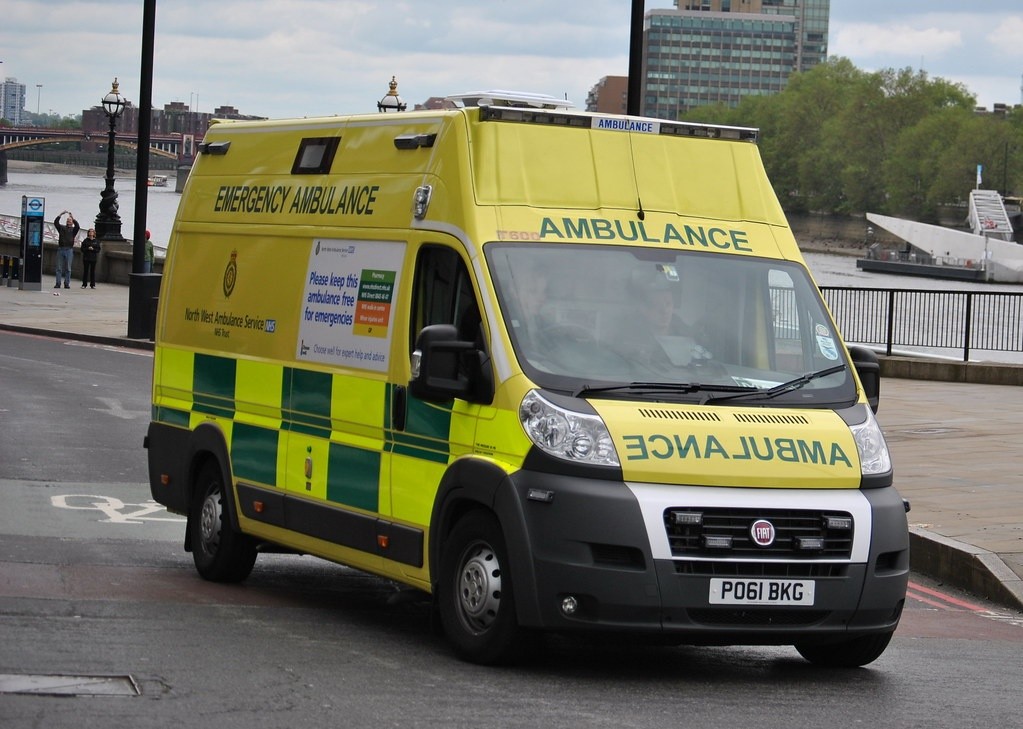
[145,231,150,239]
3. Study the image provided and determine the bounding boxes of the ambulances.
[144,105,909,665]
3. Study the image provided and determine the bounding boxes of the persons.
[54,210,79,289]
[507,260,548,318]
[81,229,101,289]
[144,231,155,274]
[644,287,674,327]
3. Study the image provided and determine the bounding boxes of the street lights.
[37,85,42,115]
[95,77,128,242]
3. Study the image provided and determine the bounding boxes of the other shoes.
[82,285,85,289]
[54,284,60,288]
[91,286,96,290]
[64,285,70,289]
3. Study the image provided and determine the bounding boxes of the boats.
[148,175,168,186]
